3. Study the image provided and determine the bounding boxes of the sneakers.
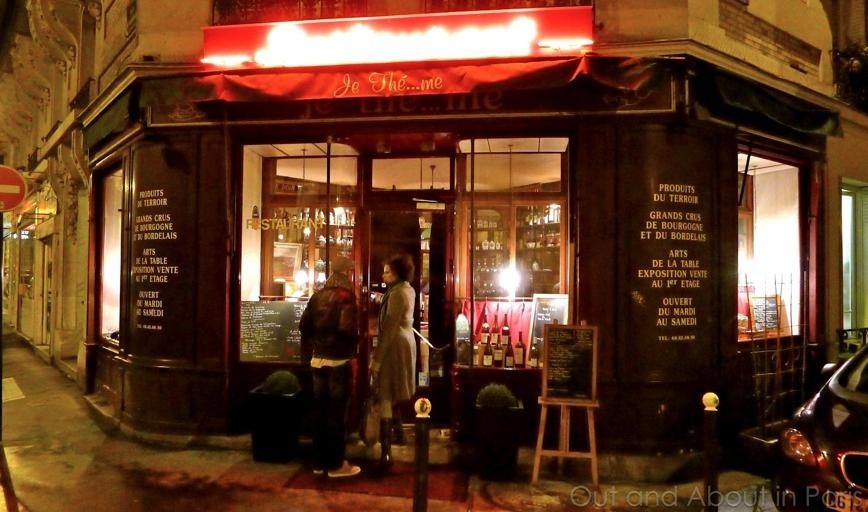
[313,460,361,478]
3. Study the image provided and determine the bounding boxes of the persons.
[367,254,417,474]
[297,255,362,478]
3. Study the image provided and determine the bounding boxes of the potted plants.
[249,370,304,464]
[474,383,524,484]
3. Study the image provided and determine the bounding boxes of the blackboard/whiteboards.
[526,293,570,368]
[542,324,598,404]
[748,294,783,332]
[240,301,309,362]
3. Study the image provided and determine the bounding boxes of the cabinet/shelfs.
[262,176,571,305]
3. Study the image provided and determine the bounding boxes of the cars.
[772,344,868,511]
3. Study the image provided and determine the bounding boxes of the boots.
[378,417,394,468]
[390,416,407,444]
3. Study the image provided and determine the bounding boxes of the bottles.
[472,213,510,301]
[478,314,527,370]
[522,204,561,271]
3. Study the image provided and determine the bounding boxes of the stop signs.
[0,164,27,212]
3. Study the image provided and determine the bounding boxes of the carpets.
[283,459,470,503]
